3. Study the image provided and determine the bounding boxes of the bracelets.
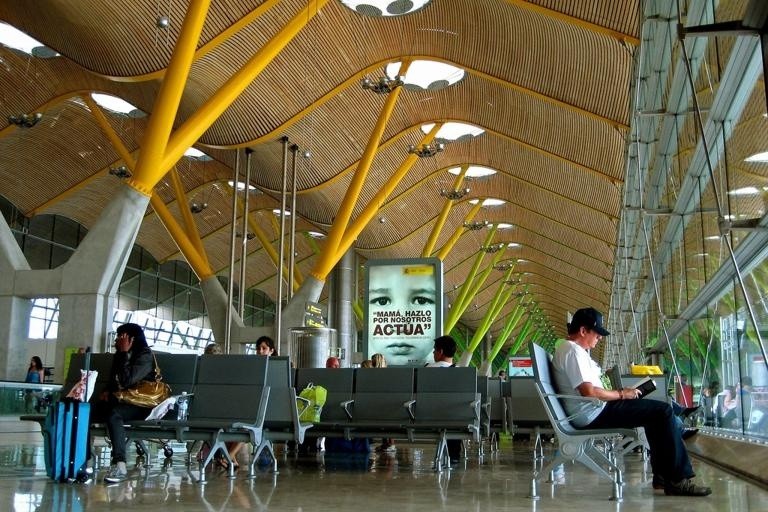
[617,389,624,400]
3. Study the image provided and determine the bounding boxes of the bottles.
[176,391,188,421]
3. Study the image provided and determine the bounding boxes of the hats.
[570,307,609,338]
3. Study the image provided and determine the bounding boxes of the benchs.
[260,349,556,473]
[529,340,639,499]
[42,353,271,486]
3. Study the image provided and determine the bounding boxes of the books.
[632,376,656,399]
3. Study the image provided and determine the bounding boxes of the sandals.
[219,459,229,469]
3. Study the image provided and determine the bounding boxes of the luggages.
[41,352,94,486]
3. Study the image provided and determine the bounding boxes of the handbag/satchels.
[112,348,174,409]
[146,391,195,420]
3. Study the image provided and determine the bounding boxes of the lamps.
[93,94,139,180]
[0,49,65,129]
[183,148,220,216]
[340,0,527,270]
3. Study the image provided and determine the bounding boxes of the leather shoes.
[664,478,712,496]
[683,404,702,418]
[104,466,129,483]
[682,429,700,441]
[652,475,665,489]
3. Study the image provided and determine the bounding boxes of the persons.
[498,370,506,382]
[370,354,397,451]
[426,336,459,368]
[204,344,223,354]
[361,360,374,368]
[218,336,278,468]
[367,264,437,367]
[676,372,754,430]
[78,321,160,484]
[326,357,341,368]
[550,307,712,497]
[24,354,44,386]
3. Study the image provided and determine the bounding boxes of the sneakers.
[375,444,386,452]
[383,445,397,452]
